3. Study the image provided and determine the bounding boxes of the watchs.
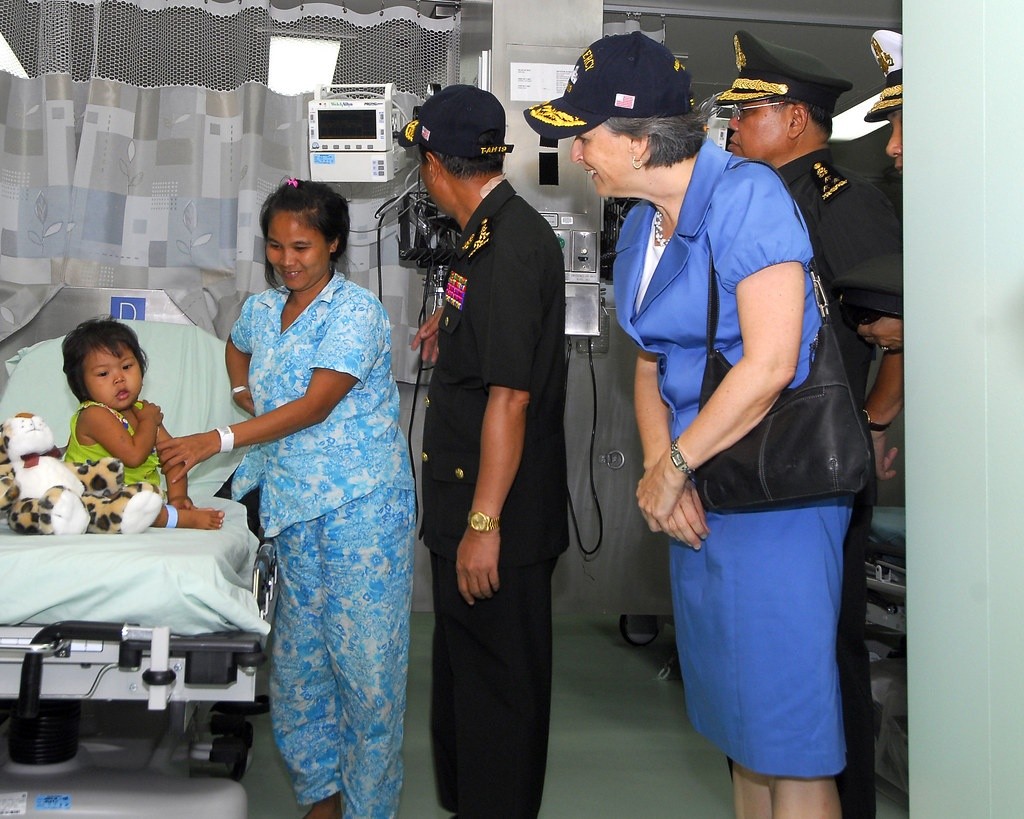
[467,511,501,530]
[862,409,891,431]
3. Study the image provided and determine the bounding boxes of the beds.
[1,319,279,819]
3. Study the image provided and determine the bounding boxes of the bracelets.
[215,425,234,453]
[231,385,247,398]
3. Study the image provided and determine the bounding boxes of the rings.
[880,346,890,351]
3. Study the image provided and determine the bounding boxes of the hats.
[717,29,854,108]
[864,30,902,123]
[398,84,515,156]
[522,31,694,140]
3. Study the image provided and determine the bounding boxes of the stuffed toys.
[0,413,162,537]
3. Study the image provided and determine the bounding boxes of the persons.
[858,30,903,351]
[714,31,904,818]
[63,314,224,528]
[522,33,855,819]
[398,84,566,819]
[157,175,416,819]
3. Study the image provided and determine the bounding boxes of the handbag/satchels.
[694,316,876,515]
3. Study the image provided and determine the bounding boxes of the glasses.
[731,100,800,122]
